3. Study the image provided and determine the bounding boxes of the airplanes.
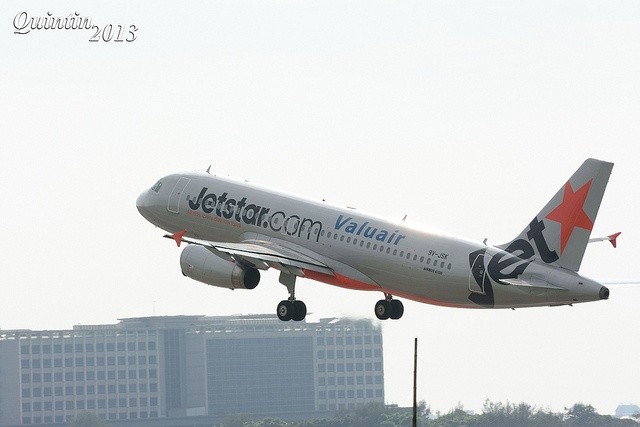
[137,157,621,321]
